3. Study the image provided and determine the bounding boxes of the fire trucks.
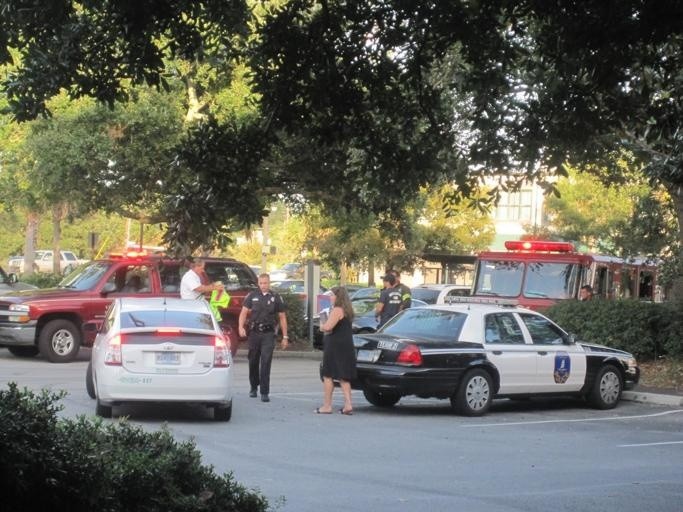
[467,241,667,309]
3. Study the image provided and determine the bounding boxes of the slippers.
[340,409,352,415]
[313,408,332,414]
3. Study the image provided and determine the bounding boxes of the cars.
[319,302,640,417]
[0,265,39,295]
[247,263,471,351]
[81,296,234,420]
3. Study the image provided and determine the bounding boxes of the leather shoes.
[249,387,257,397]
[261,394,269,402]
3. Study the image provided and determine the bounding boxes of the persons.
[238,273,288,401]
[381,269,411,312]
[178,257,225,301]
[311,286,356,415]
[374,273,402,330]
[580,285,593,302]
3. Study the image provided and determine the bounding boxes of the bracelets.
[282,335,289,339]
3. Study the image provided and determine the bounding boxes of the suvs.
[0,247,259,363]
[7,250,79,277]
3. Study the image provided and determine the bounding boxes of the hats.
[380,275,395,284]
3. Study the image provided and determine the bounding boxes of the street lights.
[259,202,277,273]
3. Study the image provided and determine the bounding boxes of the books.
[319,307,332,335]
